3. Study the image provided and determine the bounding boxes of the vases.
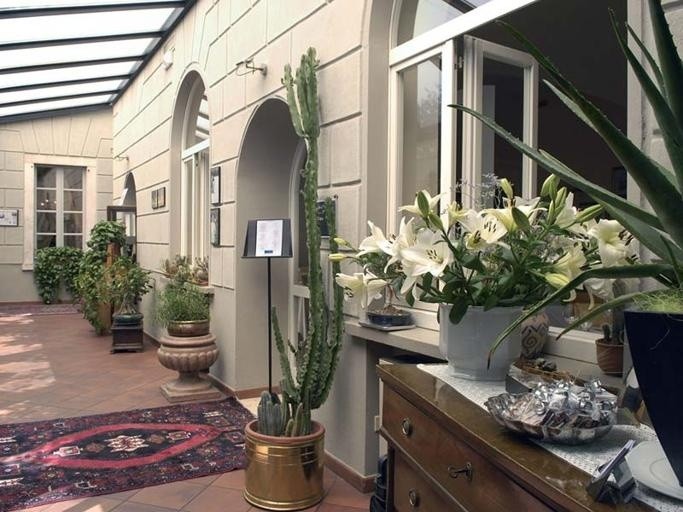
[367,311,413,328]
[440,303,522,380]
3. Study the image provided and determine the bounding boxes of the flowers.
[354,174,599,303]
[328,215,415,308]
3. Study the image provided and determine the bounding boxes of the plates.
[484,394,619,444]
[625,439,682,502]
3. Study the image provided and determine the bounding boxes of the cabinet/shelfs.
[369,361,660,512]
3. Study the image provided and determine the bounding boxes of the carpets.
[0,396,258,511]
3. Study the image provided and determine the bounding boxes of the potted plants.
[440,0,683,489]
[154,254,220,334]
[243,45,345,512]
[108,256,154,323]
[597,282,623,376]
